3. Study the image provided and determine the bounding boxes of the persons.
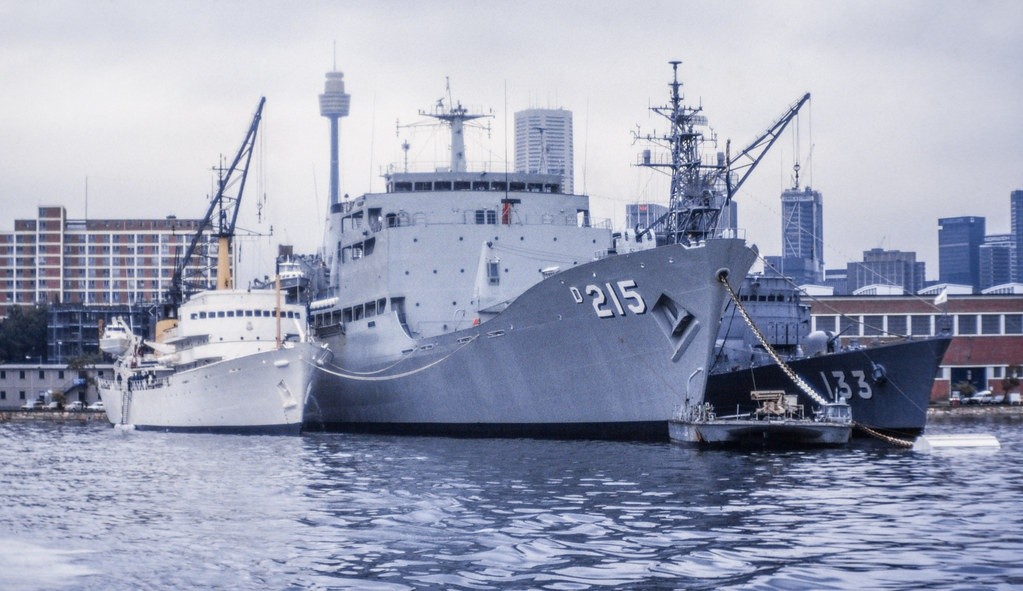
[386,212,396,227]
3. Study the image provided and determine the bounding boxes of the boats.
[98,323,133,356]
[706,325,954,456]
[85,38,855,446]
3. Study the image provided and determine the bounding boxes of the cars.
[65,400,85,412]
[86,401,106,411]
[21,400,43,412]
[43,402,65,412]
[961,389,1023,405]
